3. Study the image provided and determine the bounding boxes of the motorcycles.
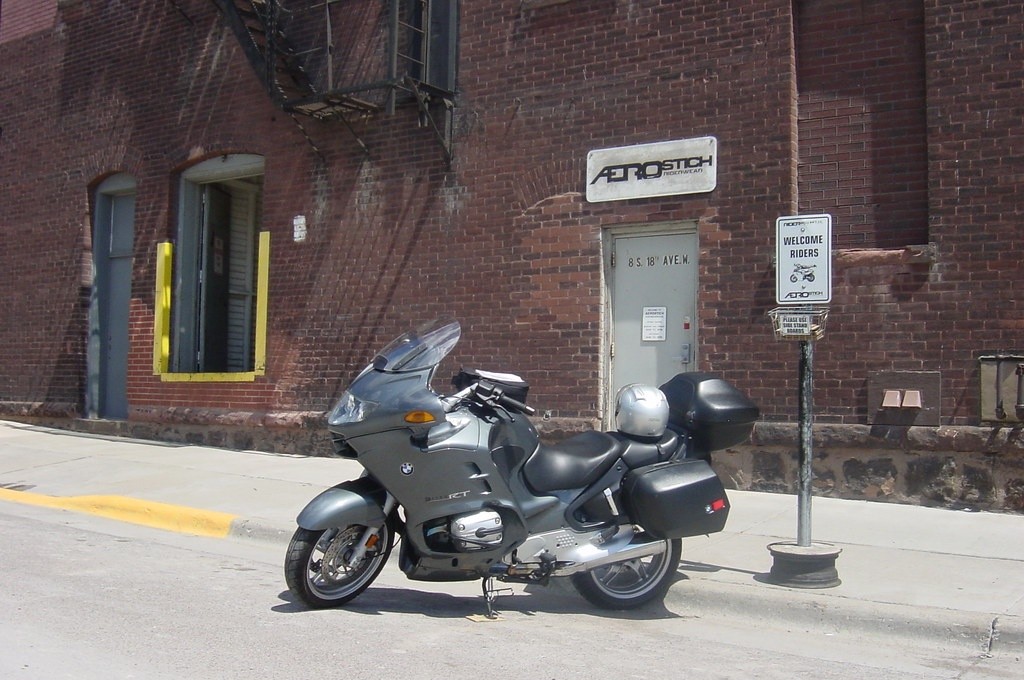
[283,316,763,611]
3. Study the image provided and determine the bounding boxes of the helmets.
[614,383,671,443]
